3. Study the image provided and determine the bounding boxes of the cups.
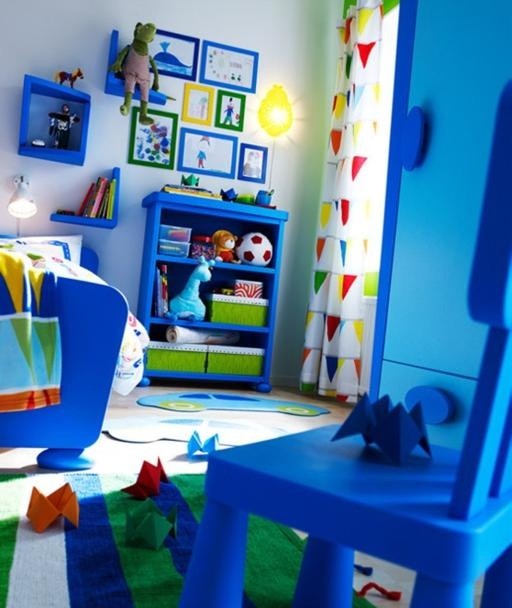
[255,191,271,205]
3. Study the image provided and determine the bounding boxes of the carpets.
[0,471,374,608]
[139,390,330,417]
[100,414,303,446]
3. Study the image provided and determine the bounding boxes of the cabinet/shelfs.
[17,73,91,166]
[368,3,512,456]
[50,167,121,229]
[136,190,289,392]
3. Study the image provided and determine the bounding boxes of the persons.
[48,103,82,152]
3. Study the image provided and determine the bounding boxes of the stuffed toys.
[110,22,166,119]
[169,253,216,322]
[213,229,240,266]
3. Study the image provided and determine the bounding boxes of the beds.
[0,234,128,471]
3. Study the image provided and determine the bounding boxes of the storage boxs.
[209,294,269,326]
[145,341,207,373]
[158,240,190,258]
[207,345,265,376]
[160,224,191,243]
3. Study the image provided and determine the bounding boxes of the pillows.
[10,233,83,266]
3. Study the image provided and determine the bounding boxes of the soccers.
[234,233,274,267]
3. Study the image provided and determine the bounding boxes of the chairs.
[179,79,512,608]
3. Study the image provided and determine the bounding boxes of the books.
[154,264,168,317]
[77,175,117,220]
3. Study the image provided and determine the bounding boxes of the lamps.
[8,172,38,237]
